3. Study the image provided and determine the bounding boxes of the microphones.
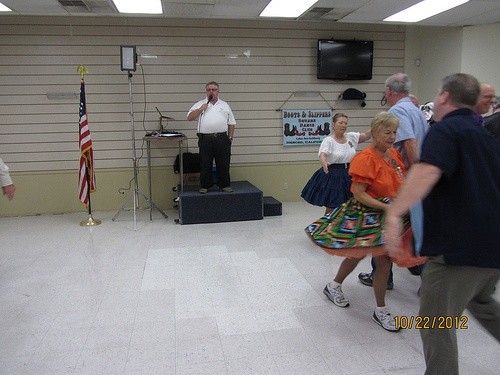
[207,95,213,105]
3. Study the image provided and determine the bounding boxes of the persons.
[304,111,408,332]
[358,74,426,295]
[474,82,500,140]
[409,94,419,107]
[382,73,500,375]
[186,81,236,192]
[301,113,372,214]
[0,159,16,201]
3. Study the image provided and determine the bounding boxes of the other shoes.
[199,188,207,193]
[219,187,233,192]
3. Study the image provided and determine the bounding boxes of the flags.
[78,81,96,209]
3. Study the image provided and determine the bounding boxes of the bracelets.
[229,137,232,140]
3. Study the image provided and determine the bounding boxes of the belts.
[202,132,226,138]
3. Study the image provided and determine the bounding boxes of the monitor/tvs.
[317,38,373,80]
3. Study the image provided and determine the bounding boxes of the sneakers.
[323,283,350,307]
[373,310,402,331]
[359,270,393,289]
